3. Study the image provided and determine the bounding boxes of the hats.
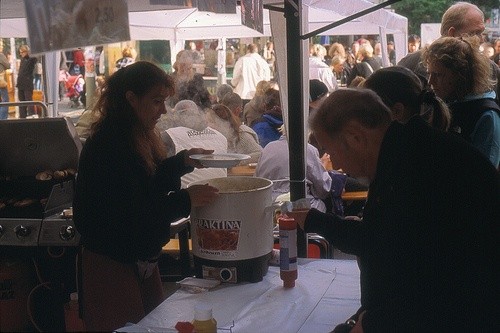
[308,79,328,103]
[175,100,199,115]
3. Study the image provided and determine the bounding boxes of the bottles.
[191,304,218,333]
[278,217,298,288]
[66,292,85,333]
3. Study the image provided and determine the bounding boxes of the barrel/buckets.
[187,175,274,284]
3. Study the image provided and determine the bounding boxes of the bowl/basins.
[189,153,251,167]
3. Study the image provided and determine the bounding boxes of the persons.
[231,43,279,99]
[397,2,500,103]
[407,34,421,54]
[71,61,219,333]
[0,33,500,333]
[158,99,229,189]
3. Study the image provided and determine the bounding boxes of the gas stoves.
[0,170,81,247]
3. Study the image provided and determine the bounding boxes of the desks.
[117,256,361,333]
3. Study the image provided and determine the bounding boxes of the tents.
[0,0,408,257]
